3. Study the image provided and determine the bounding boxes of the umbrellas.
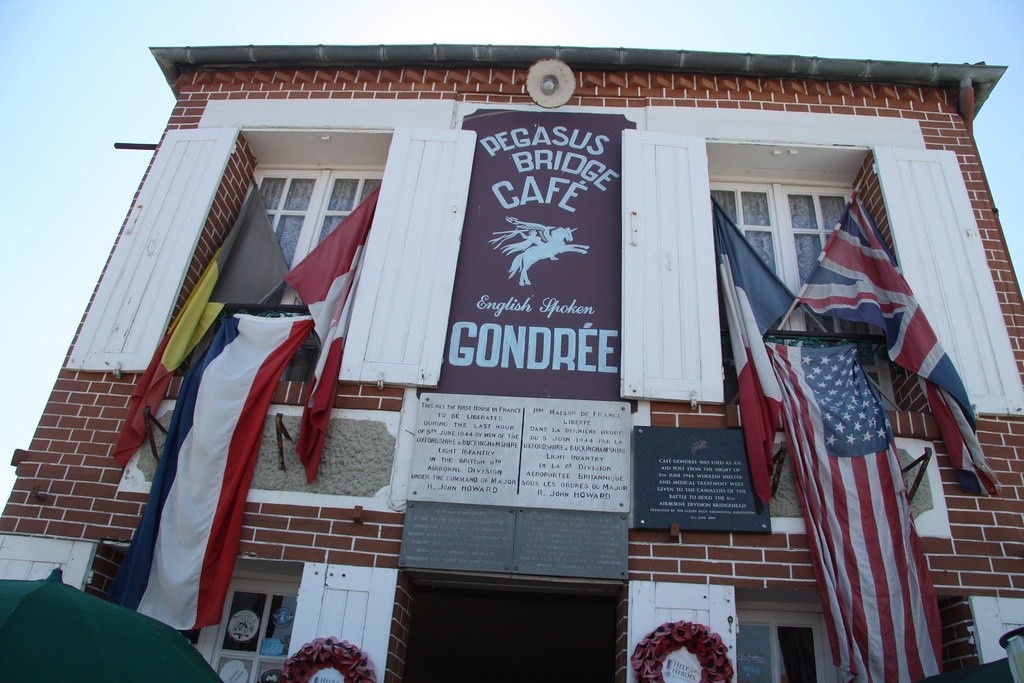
[0,561,224,683]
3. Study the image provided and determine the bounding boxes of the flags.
[713,202,796,503]
[112,188,290,466]
[108,314,315,631]
[797,188,1002,496]
[281,184,381,485]
[765,339,942,683]
